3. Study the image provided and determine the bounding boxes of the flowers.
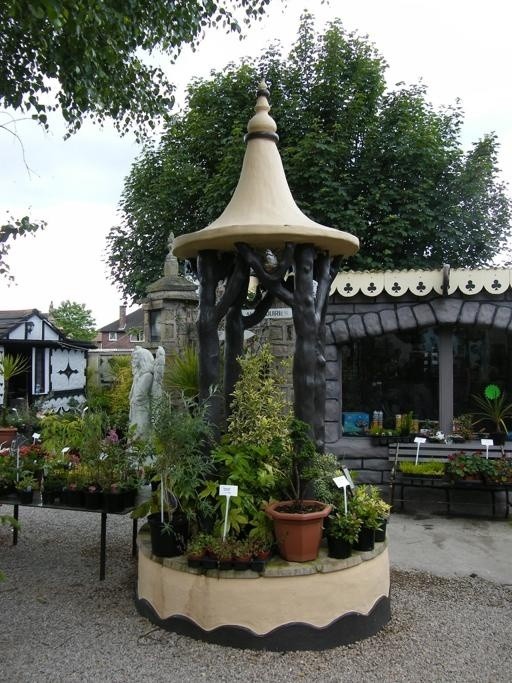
[68,426,143,491]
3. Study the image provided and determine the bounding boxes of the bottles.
[369,407,470,433]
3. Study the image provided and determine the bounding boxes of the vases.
[61,489,139,514]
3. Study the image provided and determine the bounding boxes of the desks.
[0,492,146,580]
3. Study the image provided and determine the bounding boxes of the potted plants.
[0,354,32,450]
[15,472,38,505]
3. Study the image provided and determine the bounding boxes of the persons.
[125,344,165,455]
